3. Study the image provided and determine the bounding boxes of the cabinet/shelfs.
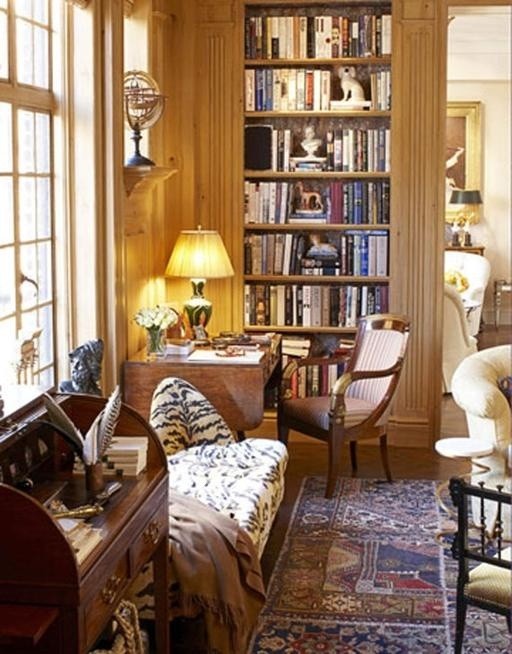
[244,0,392,419]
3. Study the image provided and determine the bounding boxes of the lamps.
[163,224,235,346]
[448,189,483,247]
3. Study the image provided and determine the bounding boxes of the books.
[244,125,391,173]
[246,14,391,59]
[245,231,388,278]
[275,335,355,400]
[244,68,390,112]
[244,179,390,225]
[74,436,148,478]
[244,282,387,329]
[57,517,102,566]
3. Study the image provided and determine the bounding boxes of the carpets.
[246,475,512,654]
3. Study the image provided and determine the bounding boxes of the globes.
[123,68,169,168]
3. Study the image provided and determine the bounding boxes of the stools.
[494,280,512,331]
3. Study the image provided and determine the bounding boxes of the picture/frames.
[444,100,483,225]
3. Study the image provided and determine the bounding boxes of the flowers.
[132,304,179,352]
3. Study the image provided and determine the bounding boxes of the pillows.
[148,376,237,456]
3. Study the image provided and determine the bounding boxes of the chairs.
[441,282,479,395]
[444,250,492,337]
[451,343,512,542]
[448,475,512,654]
[277,313,413,500]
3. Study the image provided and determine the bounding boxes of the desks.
[123,334,283,443]
[434,436,495,551]
[0,384,171,654]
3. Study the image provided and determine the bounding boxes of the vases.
[146,331,168,360]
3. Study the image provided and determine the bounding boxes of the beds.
[122,436,290,622]
